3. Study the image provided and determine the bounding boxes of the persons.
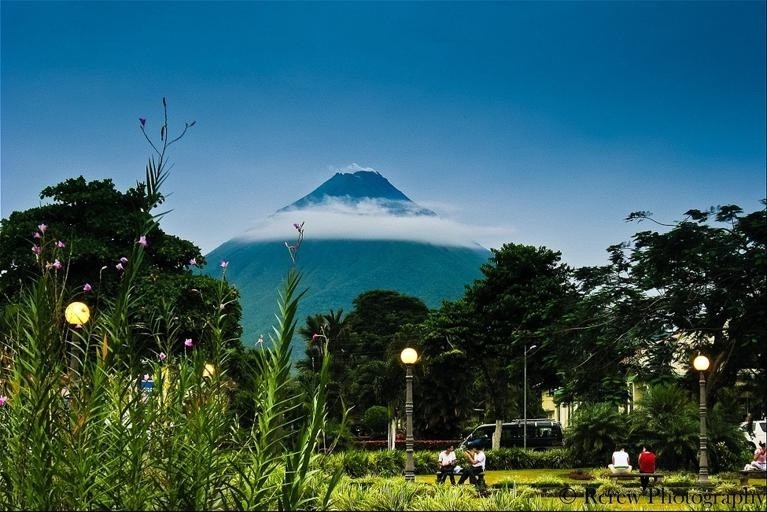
[743,443,766,471]
[607,445,633,473]
[437,443,458,486]
[456,443,487,487]
[638,443,657,495]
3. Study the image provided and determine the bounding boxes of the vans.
[459,419,563,451]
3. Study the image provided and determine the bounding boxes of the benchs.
[608,472,664,486]
[435,470,485,484]
[736,468,766,484]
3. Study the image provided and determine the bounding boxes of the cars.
[737,422,767,452]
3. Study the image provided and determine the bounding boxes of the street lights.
[523,344,536,455]
[202,363,214,416]
[694,356,710,480]
[65,303,90,387]
[400,347,416,485]
